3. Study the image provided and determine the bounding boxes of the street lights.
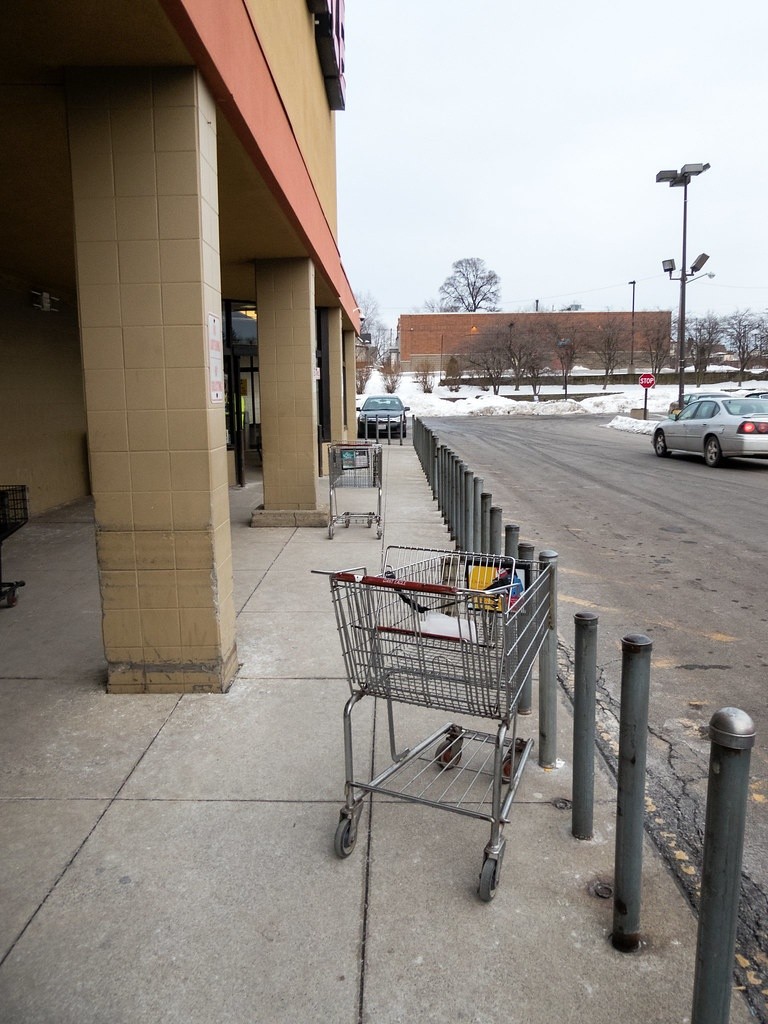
[657,162,716,410]
[675,273,716,373]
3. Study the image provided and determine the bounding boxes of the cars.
[356,394,410,439]
[745,392,768,399]
[651,398,768,468]
[667,392,732,415]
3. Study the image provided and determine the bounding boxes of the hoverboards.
[326,440,384,540]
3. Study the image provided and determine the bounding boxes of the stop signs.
[639,373,655,389]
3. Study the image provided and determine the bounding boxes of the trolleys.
[311,544,557,903]
[0,483,30,608]
